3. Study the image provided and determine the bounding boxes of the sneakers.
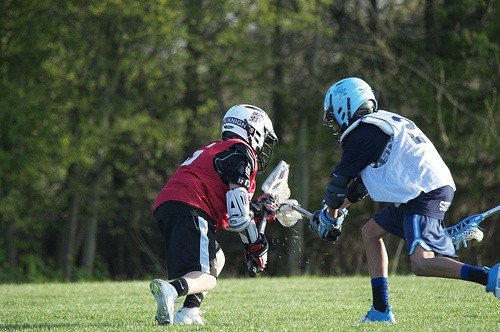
[173,306,208,326]
[482,263,500,300]
[354,306,396,323]
[150,278,179,327]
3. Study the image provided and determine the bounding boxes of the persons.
[151,103,278,327]
[307,78,500,324]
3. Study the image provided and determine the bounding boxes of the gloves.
[309,206,346,241]
[250,192,277,221]
[241,232,270,273]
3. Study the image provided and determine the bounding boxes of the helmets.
[322,76,377,142]
[222,103,279,175]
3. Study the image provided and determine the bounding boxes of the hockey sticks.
[445,205,500,245]
[275,199,313,228]
[258,160,291,234]
